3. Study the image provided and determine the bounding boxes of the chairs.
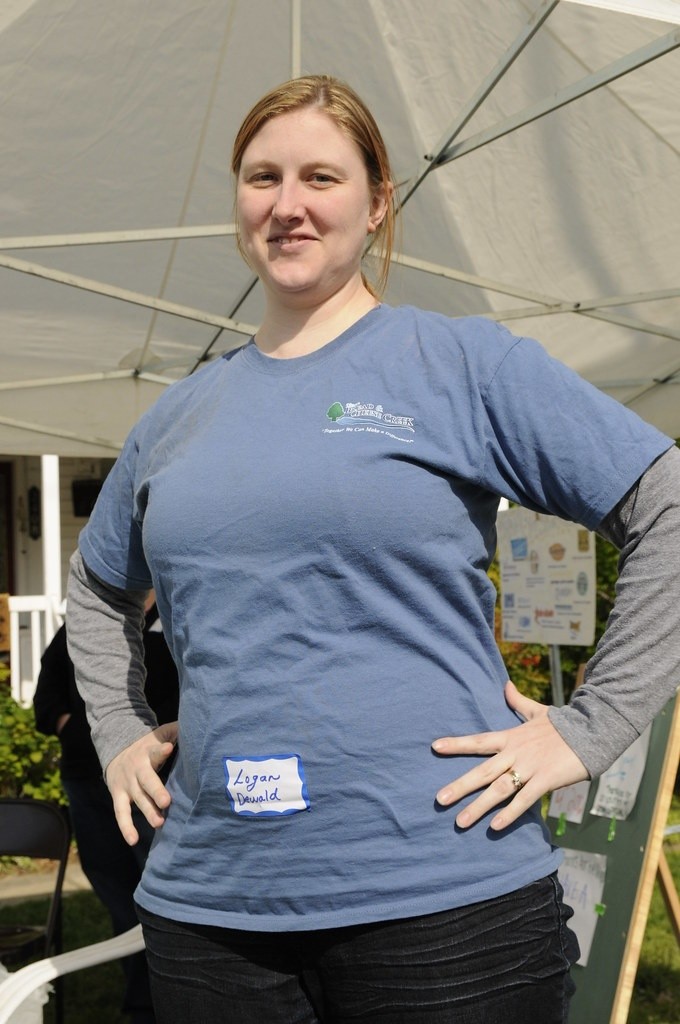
[0,797,72,1024]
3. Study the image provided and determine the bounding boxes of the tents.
[0,0,680,458]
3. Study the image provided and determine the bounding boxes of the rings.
[506,769,524,790]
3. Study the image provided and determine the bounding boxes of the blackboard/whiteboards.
[543,660,680,1024]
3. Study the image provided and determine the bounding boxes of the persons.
[32,76,680,1024]
